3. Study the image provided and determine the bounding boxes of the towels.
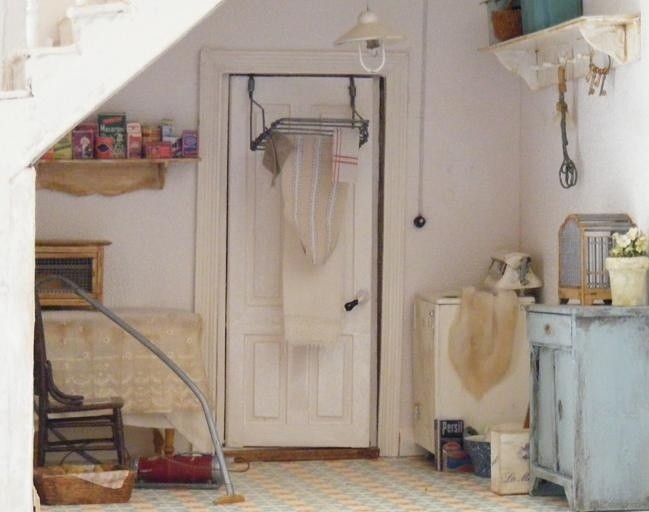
[264,128,359,267]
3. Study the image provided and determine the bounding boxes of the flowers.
[608,228,648,256]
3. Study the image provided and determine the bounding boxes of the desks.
[34,308,214,456]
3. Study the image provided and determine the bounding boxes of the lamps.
[333,0,404,74]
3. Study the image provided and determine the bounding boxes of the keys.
[585,65,608,96]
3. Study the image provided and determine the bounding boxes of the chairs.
[36,293,127,466]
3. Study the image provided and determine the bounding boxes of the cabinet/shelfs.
[525,307,649,511]
